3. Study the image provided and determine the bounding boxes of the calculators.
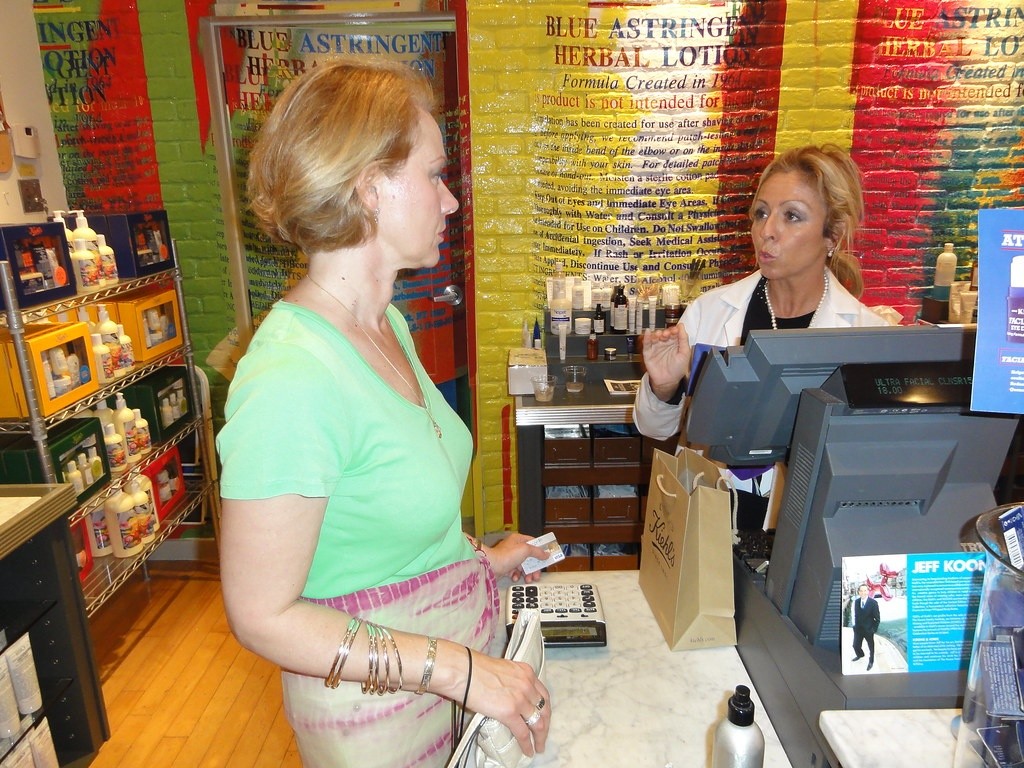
[505,583,607,648]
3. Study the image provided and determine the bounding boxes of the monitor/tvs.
[686,323,980,466]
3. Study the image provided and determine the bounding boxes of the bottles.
[932,243,956,300]
[73,232,119,293]
[105,408,151,471]
[611,285,627,333]
[710,684,765,768]
[550,262,584,334]
[91,325,135,385]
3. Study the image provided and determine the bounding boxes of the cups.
[531,365,588,402]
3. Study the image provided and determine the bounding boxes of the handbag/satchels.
[639,447,741,652]
[446,608,546,768]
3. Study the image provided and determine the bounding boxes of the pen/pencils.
[687,259,705,297]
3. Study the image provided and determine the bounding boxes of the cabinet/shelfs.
[0,239,219,624]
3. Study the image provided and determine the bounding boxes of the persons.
[634,147,892,532]
[216,56,552,768]
[852,585,880,670]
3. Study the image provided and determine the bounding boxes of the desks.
[516,386,663,575]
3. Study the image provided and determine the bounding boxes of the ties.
[862,601,865,608]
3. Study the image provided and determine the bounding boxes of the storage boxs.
[0,208,195,583]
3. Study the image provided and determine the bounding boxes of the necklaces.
[764,274,828,330]
[306,274,442,438]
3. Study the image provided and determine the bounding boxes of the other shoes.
[867,659,874,670]
[852,653,864,661]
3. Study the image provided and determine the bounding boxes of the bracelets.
[325,616,437,695]
[478,538,481,550]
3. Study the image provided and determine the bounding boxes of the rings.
[536,694,545,710]
[526,706,541,725]
